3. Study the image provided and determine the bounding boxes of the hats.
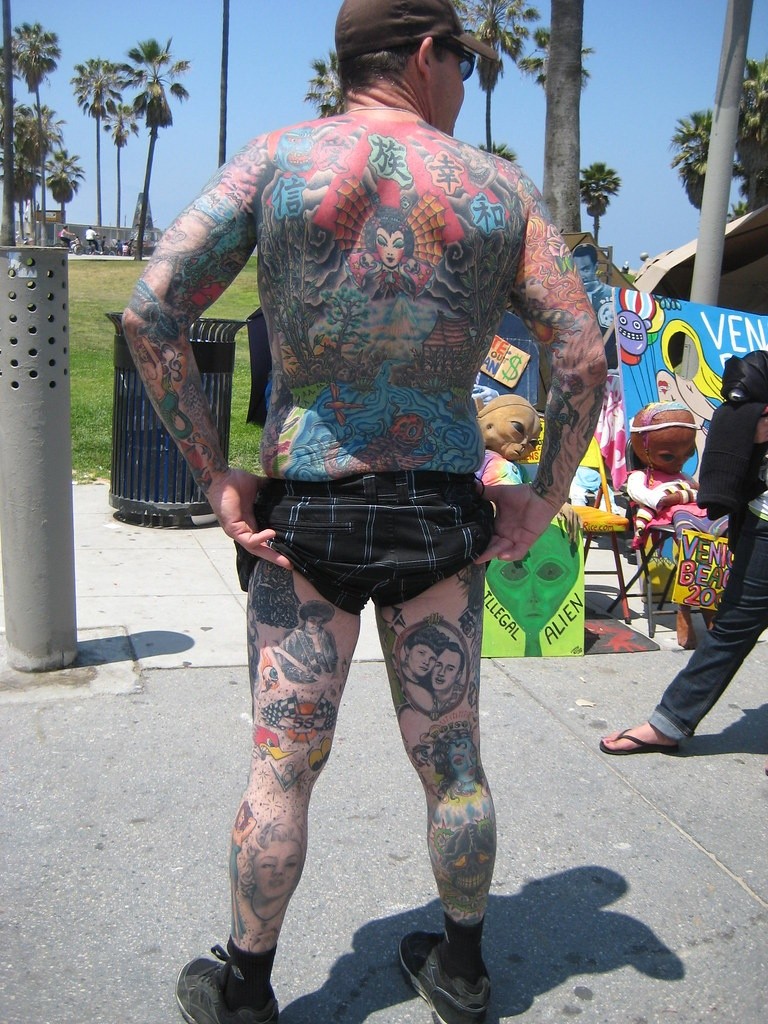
[335,0,498,62]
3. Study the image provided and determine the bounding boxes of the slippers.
[600,729,678,754]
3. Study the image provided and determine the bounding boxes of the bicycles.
[52,236,117,256]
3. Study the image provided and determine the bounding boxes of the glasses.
[433,38,476,80]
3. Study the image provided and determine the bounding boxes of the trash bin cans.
[104,310,249,529]
[0,244,81,672]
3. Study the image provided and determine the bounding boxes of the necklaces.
[345,107,414,116]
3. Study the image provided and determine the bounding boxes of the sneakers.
[398,930,490,1024]
[175,945,280,1024]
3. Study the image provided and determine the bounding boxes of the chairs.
[605,437,705,638]
[562,435,632,624]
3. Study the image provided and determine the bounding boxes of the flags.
[593,375,628,490]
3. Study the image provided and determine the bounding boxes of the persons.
[60,225,75,253]
[122,2,607,1024]
[599,350,768,780]
[625,402,729,538]
[112,237,131,256]
[85,225,107,256]
[472,394,583,542]
[70,236,82,255]
